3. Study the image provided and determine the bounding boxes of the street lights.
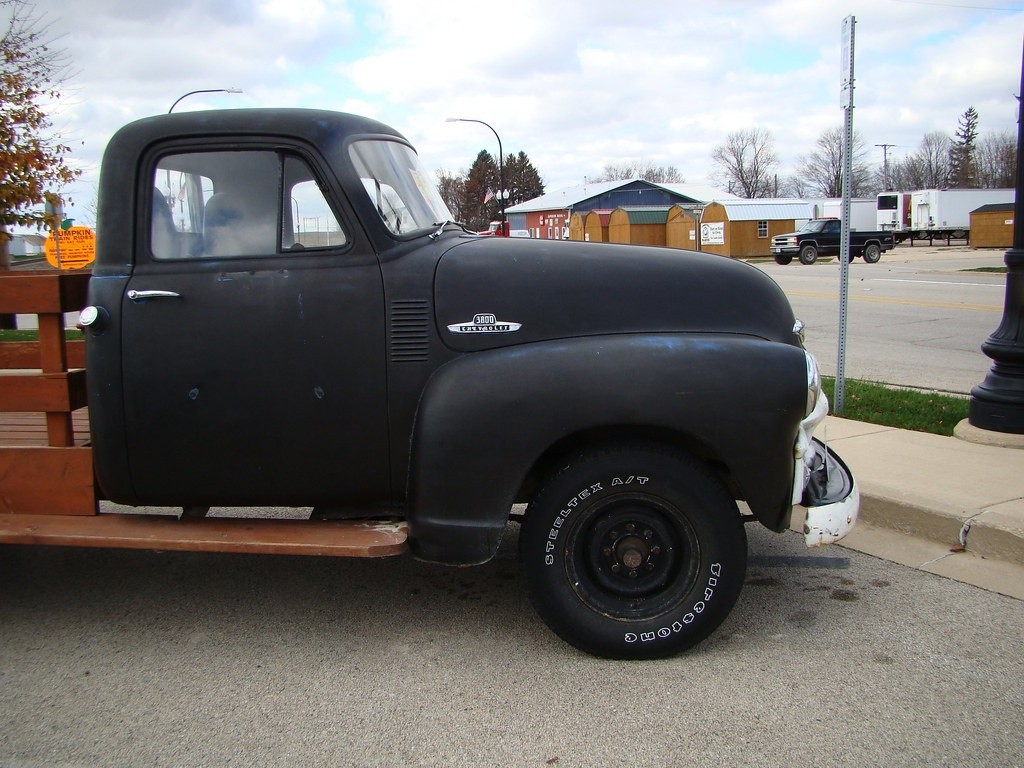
[168,89,243,213]
[444,118,506,237]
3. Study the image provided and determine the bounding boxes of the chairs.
[150,185,180,260]
[202,192,262,257]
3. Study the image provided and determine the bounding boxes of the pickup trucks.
[769,217,896,266]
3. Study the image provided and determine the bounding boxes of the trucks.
[0,107,858,661]
